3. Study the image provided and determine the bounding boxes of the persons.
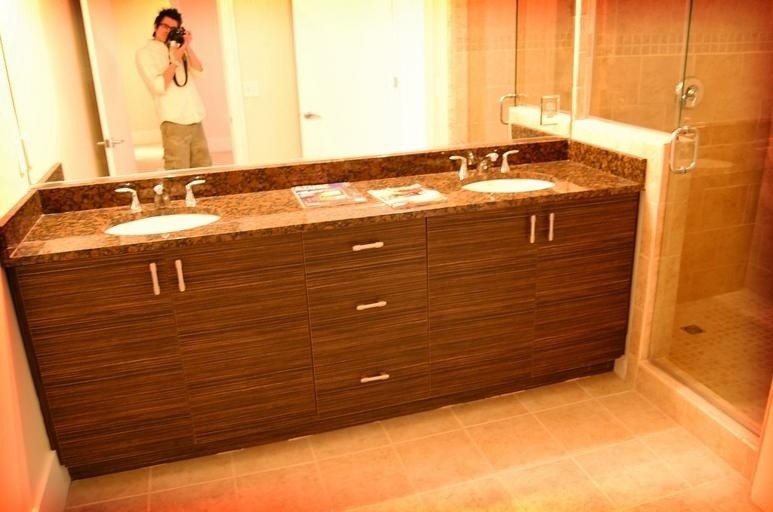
[137,7,216,171]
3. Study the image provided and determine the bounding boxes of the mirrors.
[4,0,582,187]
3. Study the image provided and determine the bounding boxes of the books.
[366,183,448,210]
[290,181,367,209]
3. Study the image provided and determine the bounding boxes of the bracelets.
[170,59,181,66]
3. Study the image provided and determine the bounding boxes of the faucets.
[475,152,500,176]
[153,184,172,208]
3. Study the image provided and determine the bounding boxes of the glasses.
[158,23,175,31]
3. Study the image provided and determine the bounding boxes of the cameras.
[165,26,186,46]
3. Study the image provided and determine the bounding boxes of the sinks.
[460,176,557,194]
[103,212,221,237]
